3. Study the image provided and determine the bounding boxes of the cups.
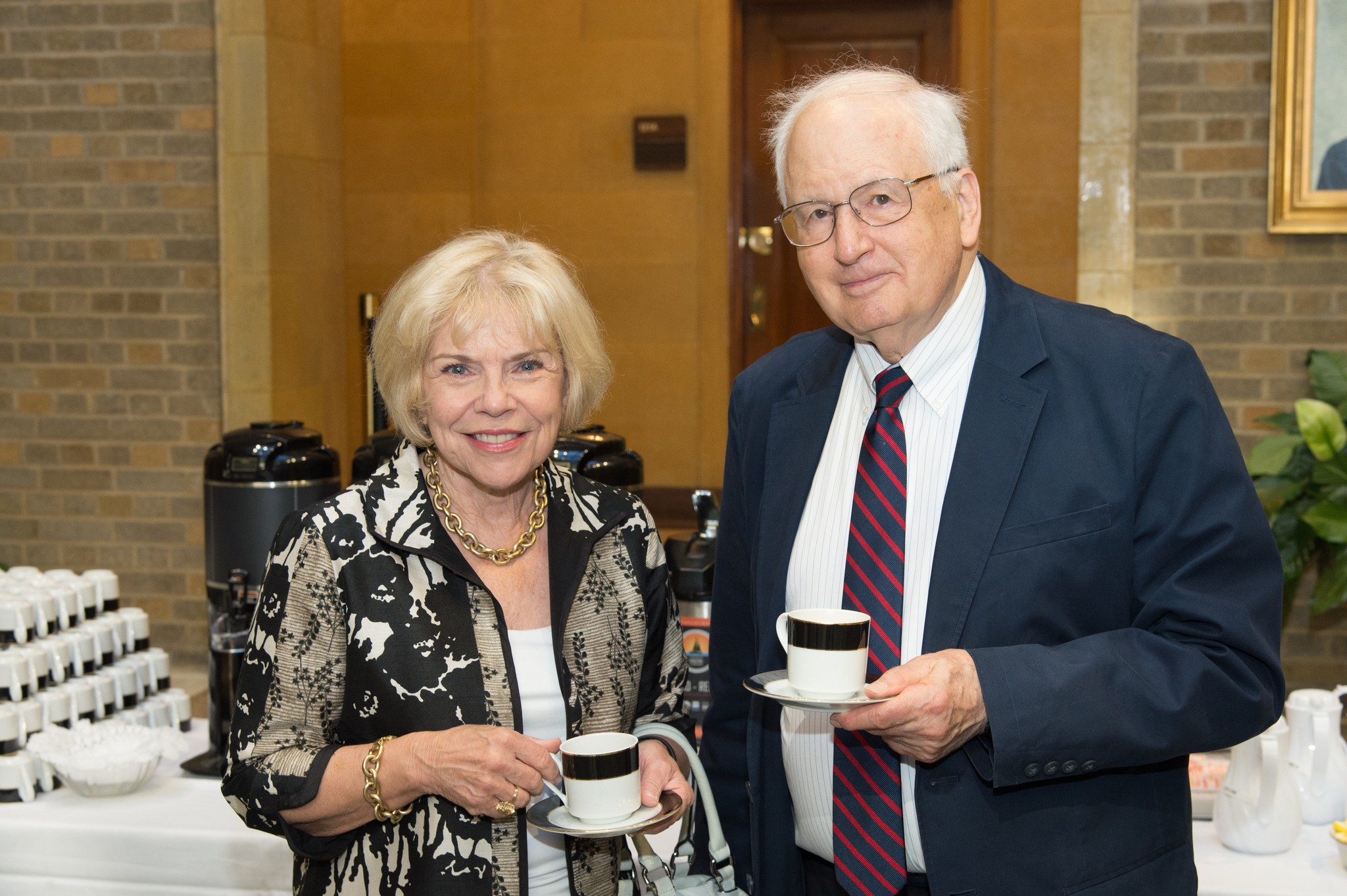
[542,732,642,825]
[0,566,192,803]
[776,608,871,700]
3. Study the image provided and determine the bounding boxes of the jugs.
[1284,688,1347,826]
[1213,715,1300,855]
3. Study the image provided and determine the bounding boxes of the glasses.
[773,167,960,248]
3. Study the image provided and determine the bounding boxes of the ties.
[833,367,914,896]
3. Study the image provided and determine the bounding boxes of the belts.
[797,846,928,889]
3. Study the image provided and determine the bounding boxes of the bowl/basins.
[1189,749,1230,820]
[40,725,161,797]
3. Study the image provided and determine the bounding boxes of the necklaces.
[422,446,548,565]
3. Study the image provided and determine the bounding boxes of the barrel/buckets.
[550,422,645,498]
[352,428,403,483]
[660,490,717,749]
[201,417,341,753]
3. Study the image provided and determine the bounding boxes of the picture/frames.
[1265,0,1347,239]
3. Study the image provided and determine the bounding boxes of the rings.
[495,784,518,816]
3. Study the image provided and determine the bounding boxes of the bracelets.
[361,735,415,824]
[636,735,677,762]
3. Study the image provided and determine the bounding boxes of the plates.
[526,790,683,839]
[742,669,899,713]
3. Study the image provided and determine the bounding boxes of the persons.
[221,232,699,896]
[697,67,1286,896]
